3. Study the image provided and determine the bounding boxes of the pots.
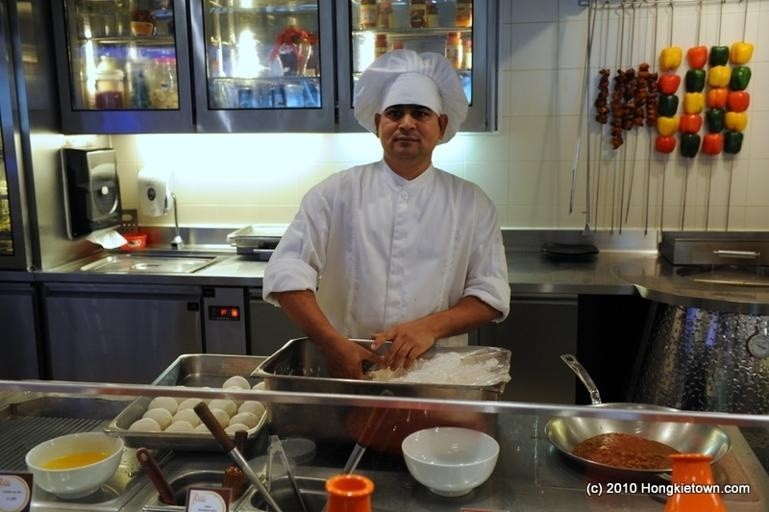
[546,353,731,481]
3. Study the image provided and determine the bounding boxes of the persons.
[261,48,512,378]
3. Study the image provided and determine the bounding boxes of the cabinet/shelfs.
[323,474,375,510]
[49,0,500,136]
[663,451,726,511]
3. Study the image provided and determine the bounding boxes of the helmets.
[540,241,600,256]
[543,254,598,264]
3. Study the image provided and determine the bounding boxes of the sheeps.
[162,190,184,250]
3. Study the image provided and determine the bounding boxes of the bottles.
[323,474,375,510]
[63,3,473,108]
[353,50,468,143]
[663,451,726,511]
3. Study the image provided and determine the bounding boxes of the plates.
[543,254,598,264]
[540,241,600,256]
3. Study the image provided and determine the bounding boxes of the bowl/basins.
[23,428,123,500]
[116,233,151,252]
[398,424,501,500]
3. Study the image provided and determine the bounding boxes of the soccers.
[656,43,755,158]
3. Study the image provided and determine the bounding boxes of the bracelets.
[398,424,501,500]
[116,233,151,252]
[23,428,123,500]
